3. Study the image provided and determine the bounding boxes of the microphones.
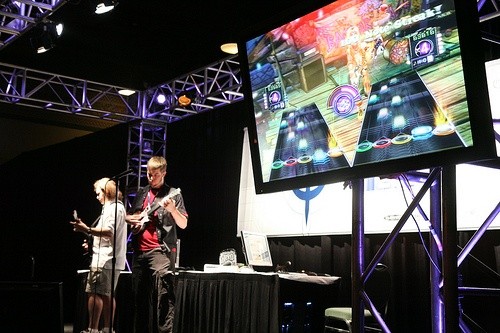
[111,169,133,180]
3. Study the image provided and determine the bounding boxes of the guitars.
[129,188,181,236]
[72,210,95,261]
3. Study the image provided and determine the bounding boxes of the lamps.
[178,90,192,105]
[94,0,118,14]
[37,18,65,53]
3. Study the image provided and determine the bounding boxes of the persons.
[125,156,187,333]
[70,177,128,333]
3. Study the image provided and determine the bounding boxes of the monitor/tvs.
[237,0,496,195]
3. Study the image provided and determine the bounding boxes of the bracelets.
[88,228,91,234]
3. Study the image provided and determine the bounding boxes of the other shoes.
[99,328,116,333]
[80,328,99,333]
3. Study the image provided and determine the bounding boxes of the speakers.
[0,281,63,333]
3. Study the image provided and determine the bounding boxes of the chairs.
[325,263,391,333]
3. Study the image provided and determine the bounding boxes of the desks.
[149,270,341,333]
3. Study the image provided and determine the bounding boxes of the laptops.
[241,230,297,271]
